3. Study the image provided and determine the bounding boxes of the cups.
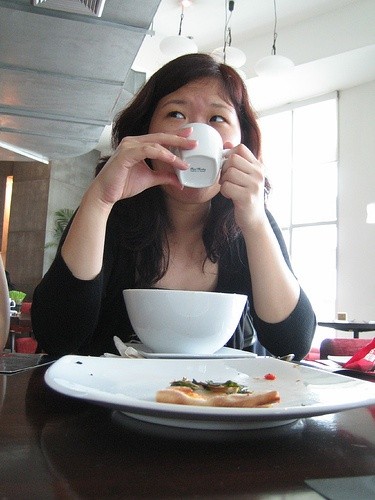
[9,298,15,310]
[168,123,232,188]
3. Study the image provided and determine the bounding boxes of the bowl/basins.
[123,289,248,353]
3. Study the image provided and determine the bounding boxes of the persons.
[31,53,317,362]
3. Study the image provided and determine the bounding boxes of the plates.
[43,354,375,430]
[123,343,257,358]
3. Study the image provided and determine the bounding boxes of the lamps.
[253,1,294,76]
[160,0,198,60]
[209,0,247,83]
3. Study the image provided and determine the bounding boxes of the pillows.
[342,338,375,373]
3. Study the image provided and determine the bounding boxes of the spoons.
[278,354,295,361]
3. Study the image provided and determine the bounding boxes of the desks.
[0,313,375,500]
